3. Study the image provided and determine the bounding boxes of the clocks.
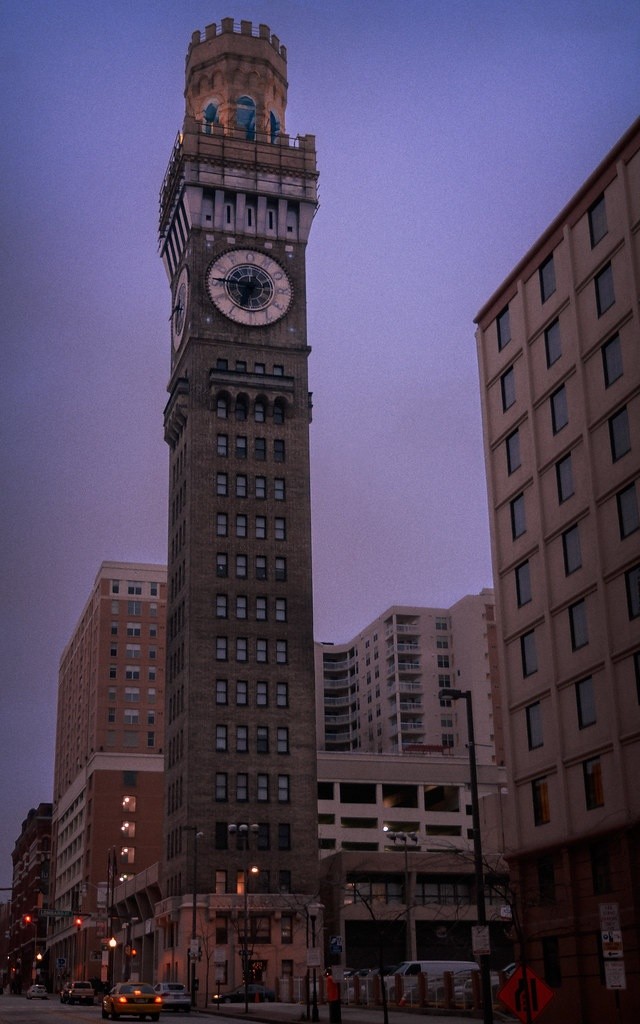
[168,264,192,353]
[203,245,294,327]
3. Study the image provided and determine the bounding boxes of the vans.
[381,961,480,1003]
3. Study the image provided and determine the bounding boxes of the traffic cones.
[397,993,407,1008]
[255,992,259,1003]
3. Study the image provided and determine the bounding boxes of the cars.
[211,984,275,1004]
[308,965,398,1001]
[102,982,163,1022]
[410,962,516,1004]
[26,984,48,1000]
[153,982,192,1013]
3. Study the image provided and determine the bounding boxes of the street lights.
[385,831,418,962]
[437,688,493,1024]
[181,824,198,1007]
[36,953,42,985]
[108,936,117,991]
[228,823,259,949]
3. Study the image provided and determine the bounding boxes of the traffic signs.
[605,961,626,990]
[601,931,623,958]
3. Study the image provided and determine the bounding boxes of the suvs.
[60,981,95,1006]
